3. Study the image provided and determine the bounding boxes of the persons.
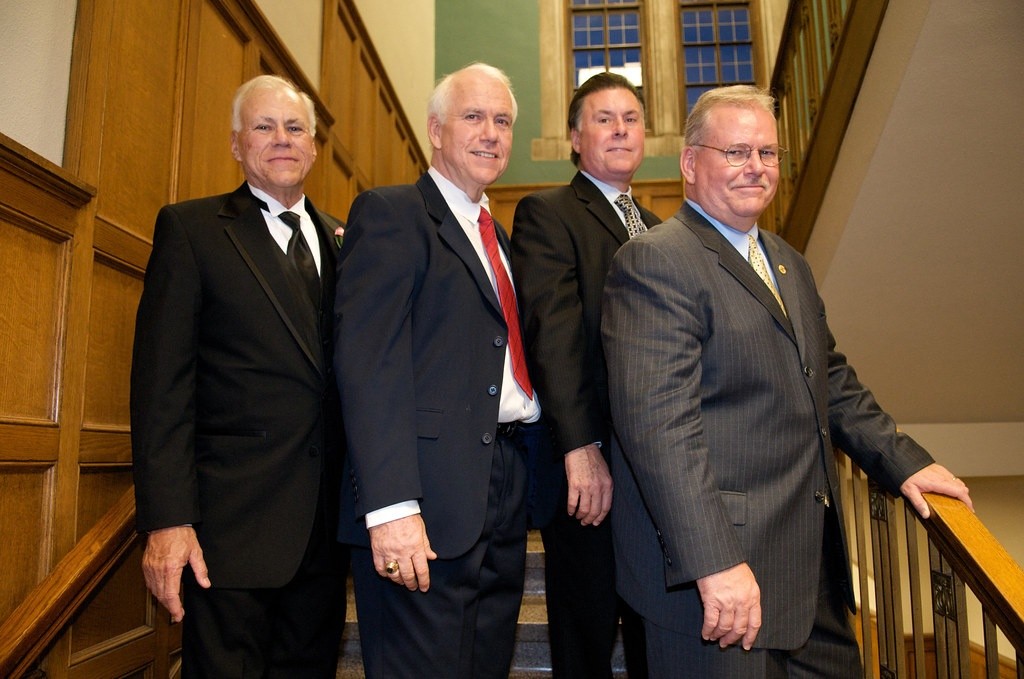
[130,76,349,679]
[336,64,541,679]
[510,72,663,679]
[603,85,975,679]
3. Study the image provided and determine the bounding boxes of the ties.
[255,198,324,346]
[615,194,650,238]
[748,235,789,321]
[477,208,535,400]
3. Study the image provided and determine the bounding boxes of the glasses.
[692,142,789,168]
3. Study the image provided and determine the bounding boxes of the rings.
[386,563,398,574]
[953,477,957,480]
